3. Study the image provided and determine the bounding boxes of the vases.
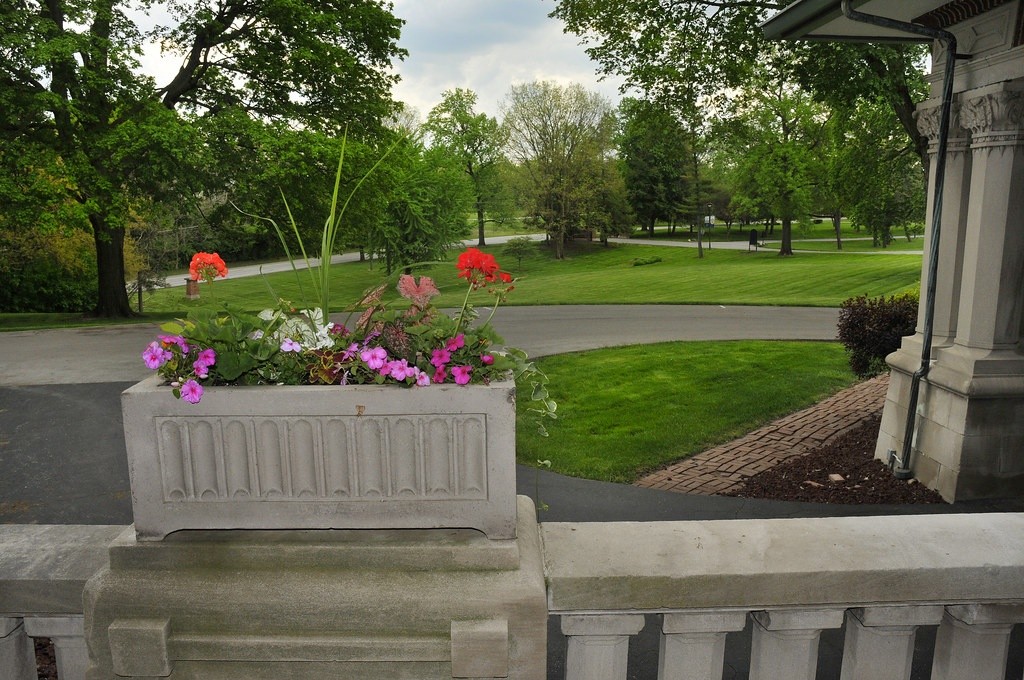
[122,372,517,540]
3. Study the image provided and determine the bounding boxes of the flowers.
[141,121,557,516]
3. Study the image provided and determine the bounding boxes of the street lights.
[707,202,712,249]
